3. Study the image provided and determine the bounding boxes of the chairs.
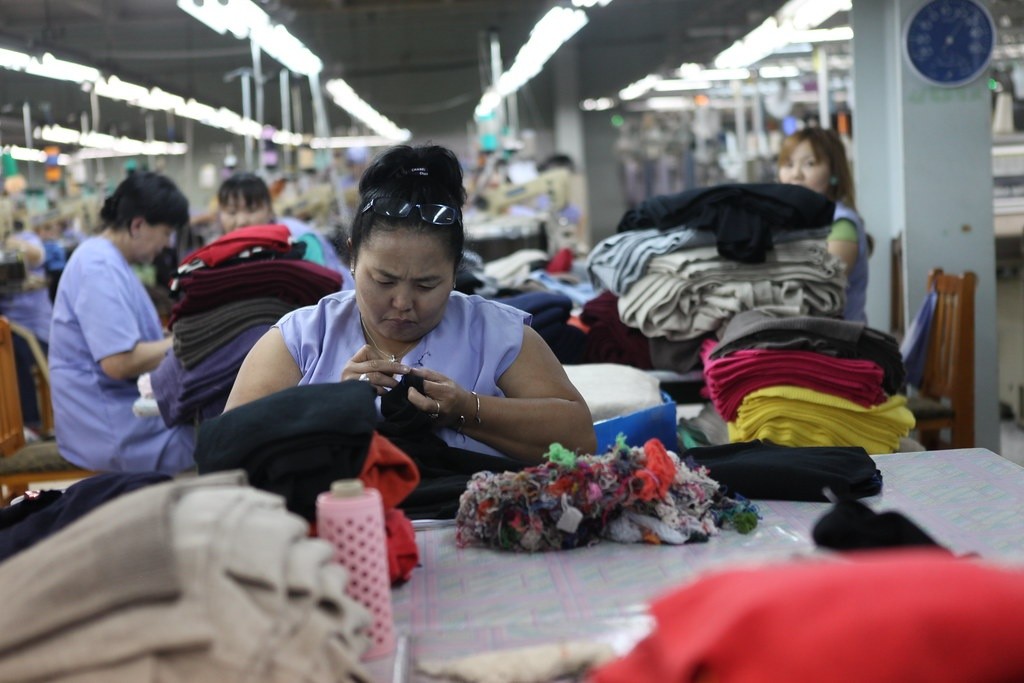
[914,269,978,448]
[0,316,107,509]
[889,234,904,344]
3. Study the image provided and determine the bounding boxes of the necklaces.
[361,320,414,363]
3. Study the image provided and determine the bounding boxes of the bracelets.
[455,390,482,434]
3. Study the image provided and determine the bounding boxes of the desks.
[386,449,1024,683]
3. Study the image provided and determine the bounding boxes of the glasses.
[361,197,461,228]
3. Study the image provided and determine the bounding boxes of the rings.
[359,373,369,383]
[428,403,440,419]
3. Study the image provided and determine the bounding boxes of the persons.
[207,172,359,290]
[777,126,870,326]
[220,144,599,465]
[0,171,197,479]
